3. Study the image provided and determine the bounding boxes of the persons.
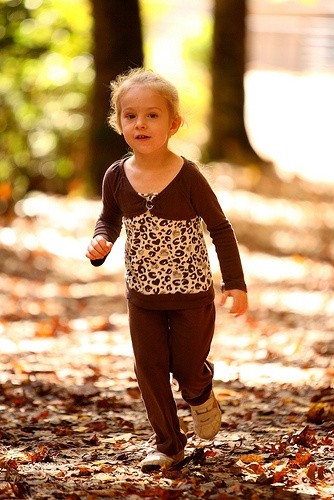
[84,66,248,470]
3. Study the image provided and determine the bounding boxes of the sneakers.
[139,445,185,472]
[189,389,222,440]
